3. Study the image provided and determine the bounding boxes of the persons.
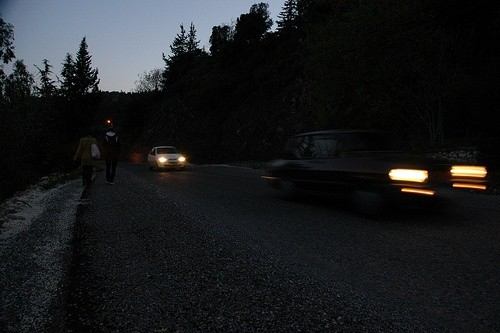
[101,121,122,186]
[72,127,101,196]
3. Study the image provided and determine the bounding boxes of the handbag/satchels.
[90,144,100,159]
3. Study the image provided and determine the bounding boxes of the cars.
[147,146,186,172]
[261,130,486,214]
